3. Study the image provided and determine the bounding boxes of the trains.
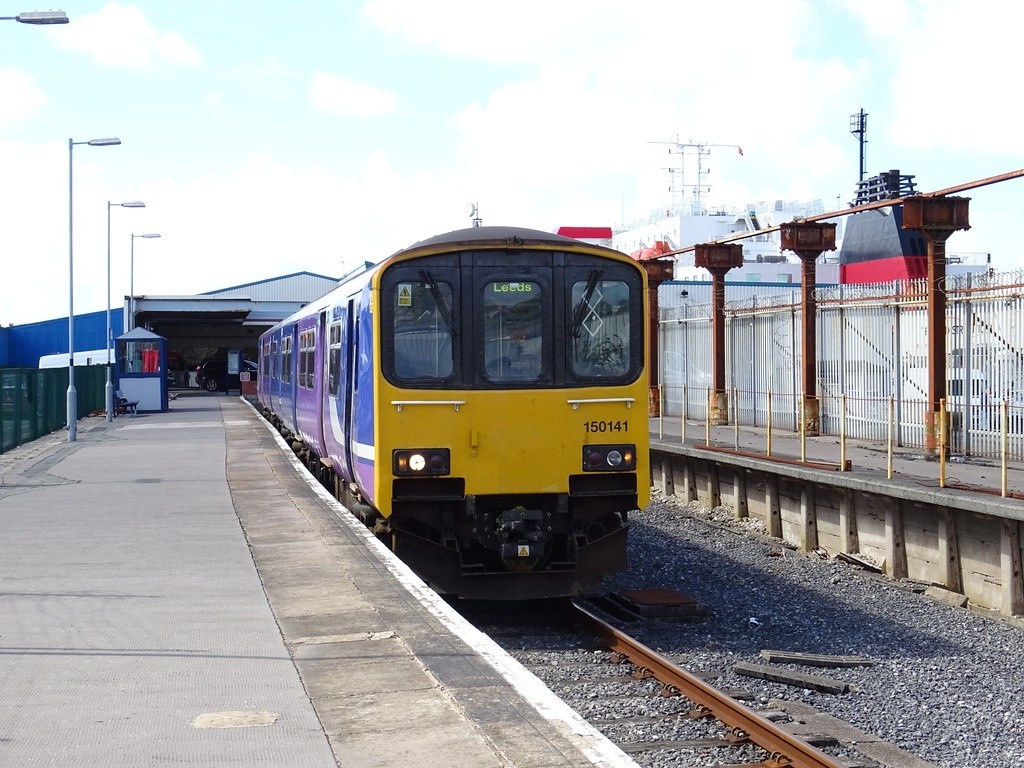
[255,224,653,601]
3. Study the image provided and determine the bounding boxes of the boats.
[601,106,997,310]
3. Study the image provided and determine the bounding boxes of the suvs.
[196,357,257,393]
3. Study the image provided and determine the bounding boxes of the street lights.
[103,202,145,423]
[62,137,123,442]
[126,233,162,371]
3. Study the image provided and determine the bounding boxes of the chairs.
[114,388,139,418]
